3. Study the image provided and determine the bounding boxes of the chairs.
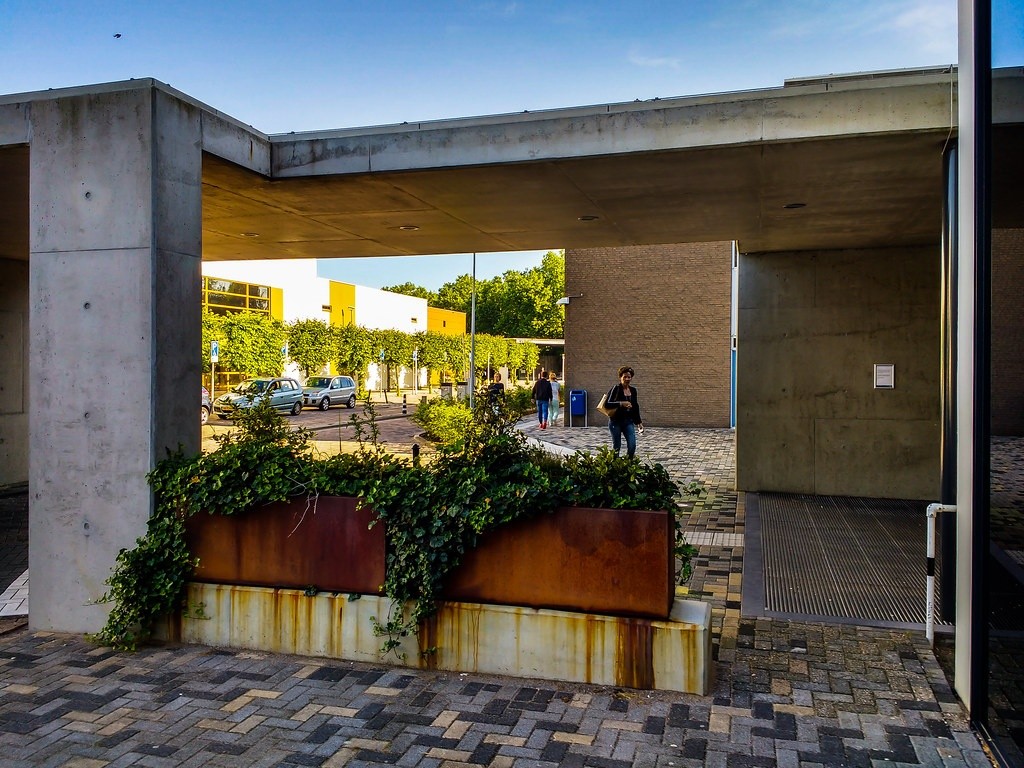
[317,380,326,387]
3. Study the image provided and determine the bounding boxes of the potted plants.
[87,395,708,652]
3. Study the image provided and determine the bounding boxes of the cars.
[212,376,305,421]
[201,385,211,425]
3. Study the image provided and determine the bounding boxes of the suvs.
[300,375,356,411]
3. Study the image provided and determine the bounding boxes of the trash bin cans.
[570,390,590,429]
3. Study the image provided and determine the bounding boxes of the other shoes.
[550,422,557,426]
[539,423,547,429]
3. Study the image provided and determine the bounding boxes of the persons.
[531,372,553,429]
[268,382,278,393]
[487,373,506,403]
[548,372,561,426]
[605,367,644,457]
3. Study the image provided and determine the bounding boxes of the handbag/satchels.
[597,384,619,417]
